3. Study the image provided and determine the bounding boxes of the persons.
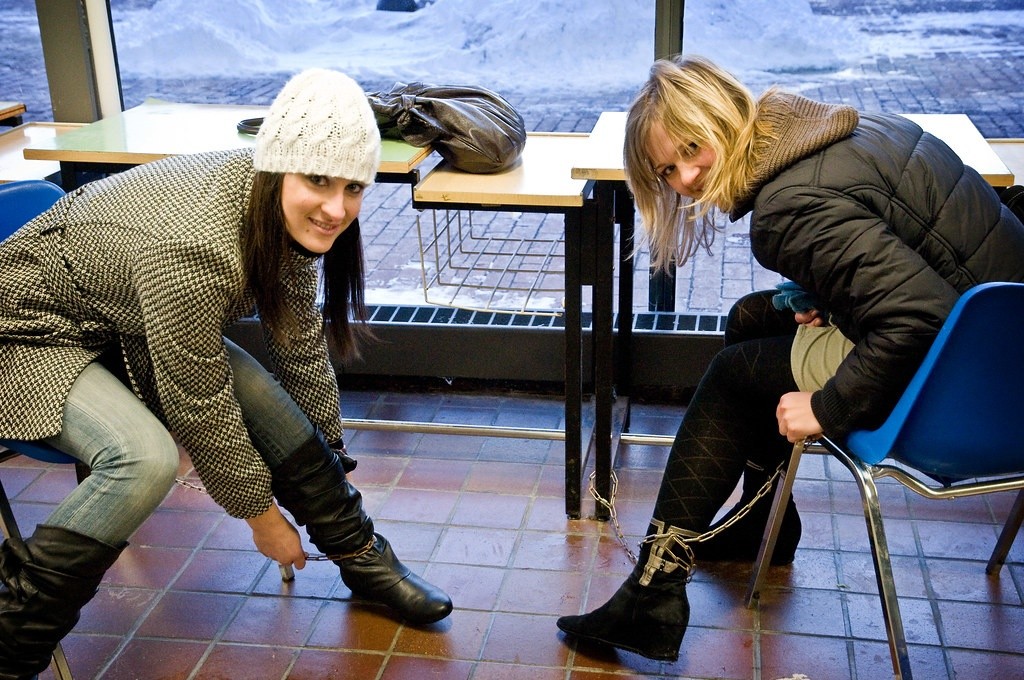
[1,69,459,680]
[557,56,1024,663]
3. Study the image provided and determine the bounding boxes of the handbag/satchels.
[236,81,527,173]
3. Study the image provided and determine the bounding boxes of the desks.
[0,99,1024,524]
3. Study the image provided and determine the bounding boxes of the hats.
[253,68,379,185]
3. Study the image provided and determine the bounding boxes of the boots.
[556,519,701,659]
[693,460,802,566]
[0,523,131,679]
[268,421,454,626]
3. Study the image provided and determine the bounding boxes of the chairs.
[0,179,90,680]
[746,282,1024,680]
